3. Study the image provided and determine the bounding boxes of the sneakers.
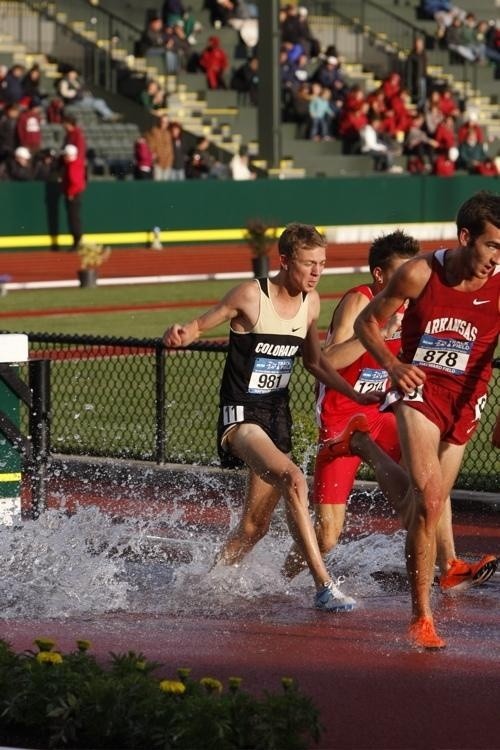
[317,413,371,466]
[438,554,498,594]
[406,616,447,650]
[314,584,357,613]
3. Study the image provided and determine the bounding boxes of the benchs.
[1,0,500,175]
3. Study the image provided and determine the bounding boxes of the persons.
[58,116,86,251]
[161,223,385,613]
[135,1,499,181]
[256,229,421,593]
[1,61,260,182]
[353,190,499,646]
[45,143,78,249]
